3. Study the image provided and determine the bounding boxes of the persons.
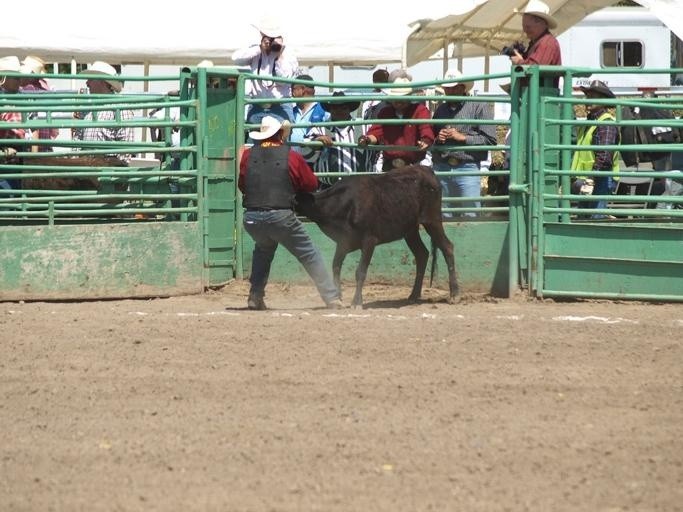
[234,115,353,312]
[0,0,681,222]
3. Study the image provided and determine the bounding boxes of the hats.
[248,115,291,140]
[435,68,474,93]
[512,0,557,29]
[295,75,314,88]
[247,18,282,39]
[0,54,48,85]
[320,92,360,113]
[579,80,616,98]
[79,60,123,93]
[379,77,413,96]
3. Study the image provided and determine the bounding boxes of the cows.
[0,156,130,217]
[294,165,460,311]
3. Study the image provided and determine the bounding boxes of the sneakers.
[326,298,347,310]
[247,291,267,311]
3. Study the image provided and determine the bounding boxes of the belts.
[392,158,418,168]
[440,156,462,166]
[248,206,277,211]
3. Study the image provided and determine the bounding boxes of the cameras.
[502,43,525,57]
[266,38,281,51]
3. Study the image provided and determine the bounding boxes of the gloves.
[579,179,596,196]
